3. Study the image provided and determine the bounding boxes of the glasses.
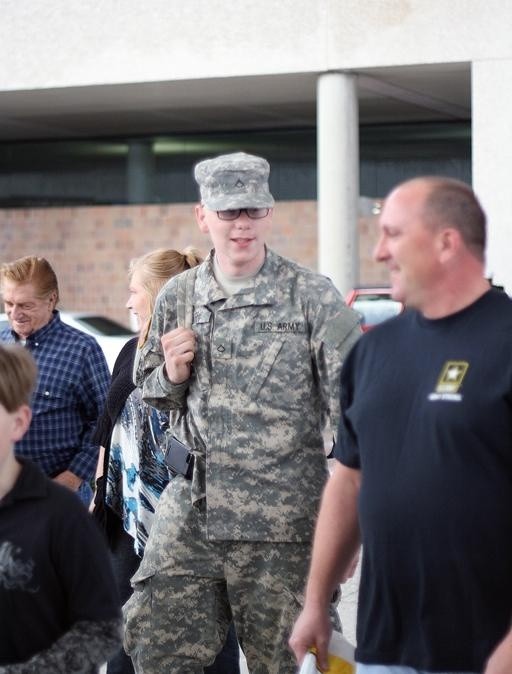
[215,208,272,220]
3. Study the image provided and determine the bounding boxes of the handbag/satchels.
[93,474,123,554]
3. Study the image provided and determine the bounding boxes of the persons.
[120,151,362,674]
[1,344,125,674]
[92,243,241,674]
[1,255,114,512]
[289,176,512,674]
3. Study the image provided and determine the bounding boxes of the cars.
[346,287,405,332]
[0,310,145,375]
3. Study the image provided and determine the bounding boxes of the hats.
[195,150,275,212]
